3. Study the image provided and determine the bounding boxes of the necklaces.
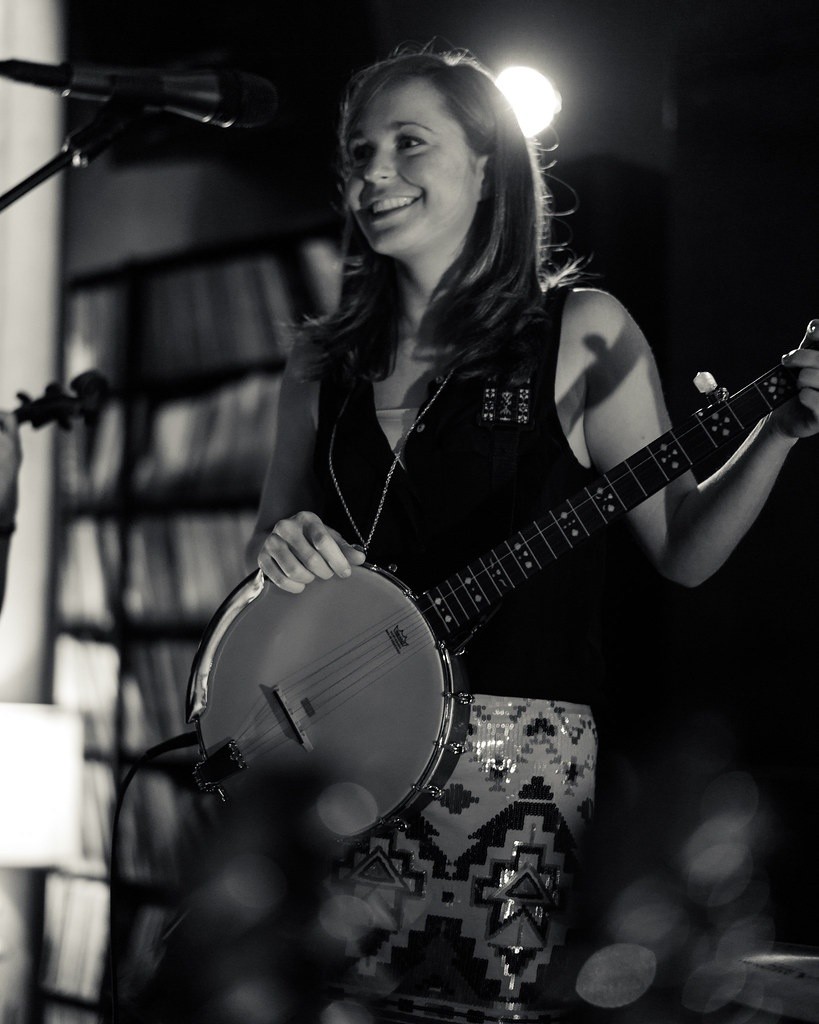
[327,370,453,554]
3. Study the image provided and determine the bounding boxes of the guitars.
[184,339,819,849]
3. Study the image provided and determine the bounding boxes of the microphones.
[0,59,277,133]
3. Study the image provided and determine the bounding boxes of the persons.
[0,411,24,617]
[243,53,819,708]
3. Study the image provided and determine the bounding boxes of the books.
[41,241,343,1024]
